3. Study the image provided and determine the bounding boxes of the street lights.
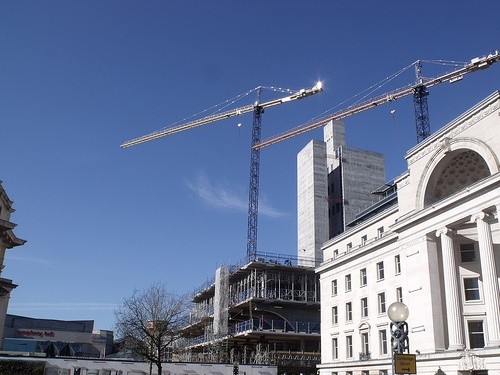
[388,301,411,375]
[233,361,240,375]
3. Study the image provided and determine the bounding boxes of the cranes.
[119,78,327,266]
[250,49,500,144]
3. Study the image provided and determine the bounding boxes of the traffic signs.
[394,355,417,375]
